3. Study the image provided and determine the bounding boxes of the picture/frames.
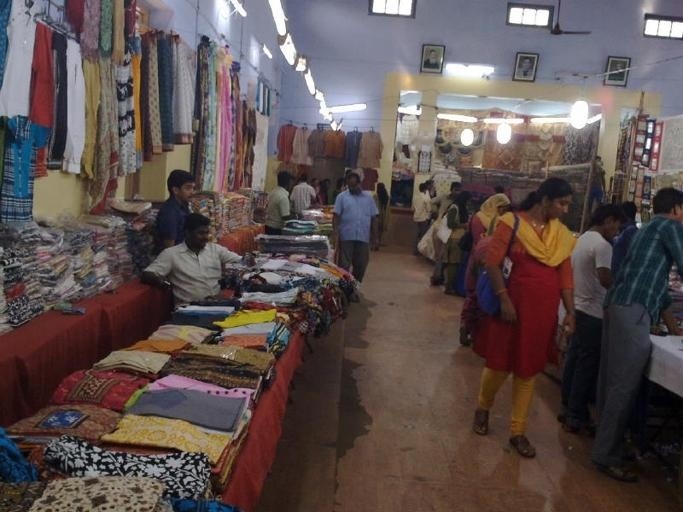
[417,42,446,75]
[603,57,632,88]
[511,50,540,83]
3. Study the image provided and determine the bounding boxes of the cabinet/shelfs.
[88,274,172,359]
[2,250,347,512]
[546,302,682,491]
[148,230,238,325]
[227,219,265,254]
[0,300,102,430]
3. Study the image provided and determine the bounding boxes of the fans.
[539,0,589,39]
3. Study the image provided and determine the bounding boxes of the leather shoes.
[555,401,647,483]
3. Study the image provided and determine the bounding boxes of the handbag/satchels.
[474,256,513,317]
[436,216,452,244]
[457,232,474,252]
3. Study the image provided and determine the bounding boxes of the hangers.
[286,121,376,138]
[34,9,66,34]
[49,13,76,39]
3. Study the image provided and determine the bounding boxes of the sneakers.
[508,433,536,457]
[472,406,489,435]
[460,326,470,346]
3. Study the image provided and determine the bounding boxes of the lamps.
[460,112,474,147]
[496,108,512,147]
[572,74,588,132]
[229,1,366,132]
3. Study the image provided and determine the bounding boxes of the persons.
[585,152,605,209]
[559,184,683,485]
[434,127,447,147]
[516,57,533,79]
[142,212,244,318]
[299,167,357,209]
[288,172,316,215]
[263,171,298,237]
[607,61,624,82]
[412,180,512,346]
[369,181,390,251]
[330,170,381,286]
[472,176,578,460]
[148,166,198,247]
[422,47,439,69]
